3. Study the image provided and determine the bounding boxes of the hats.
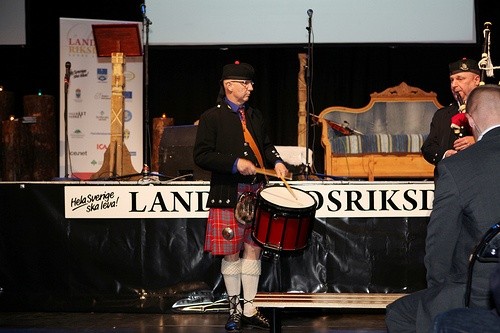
[223,61,255,80]
[449,58,480,75]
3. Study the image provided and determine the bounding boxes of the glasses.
[231,81,254,86]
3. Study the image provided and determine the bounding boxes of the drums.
[251,184,318,257]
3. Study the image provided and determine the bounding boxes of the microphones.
[65,61,71,82]
[307,9,313,28]
[140,4,146,15]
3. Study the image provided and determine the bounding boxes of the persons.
[193,61,288,333]
[386,58,500,333]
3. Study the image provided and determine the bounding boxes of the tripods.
[103,15,179,181]
[293,28,349,181]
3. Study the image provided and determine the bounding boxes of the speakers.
[158,125,214,182]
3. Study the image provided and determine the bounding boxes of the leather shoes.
[242,299,270,330]
[226,295,242,333]
